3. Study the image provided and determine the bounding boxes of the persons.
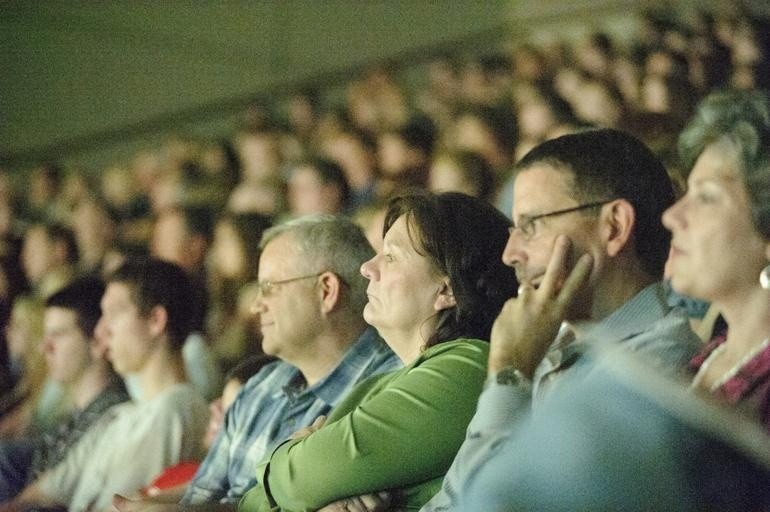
[416,129,707,512]
[0,271,129,498]
[660,88,770,512]
[237,189,518,512]
[3,257,212,512]
[4,295,47,441]
[109,212,405,512]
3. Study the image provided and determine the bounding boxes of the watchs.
[480,364,534,389]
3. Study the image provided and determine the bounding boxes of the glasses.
[505,200,613,235]
[256,271,353,298]
